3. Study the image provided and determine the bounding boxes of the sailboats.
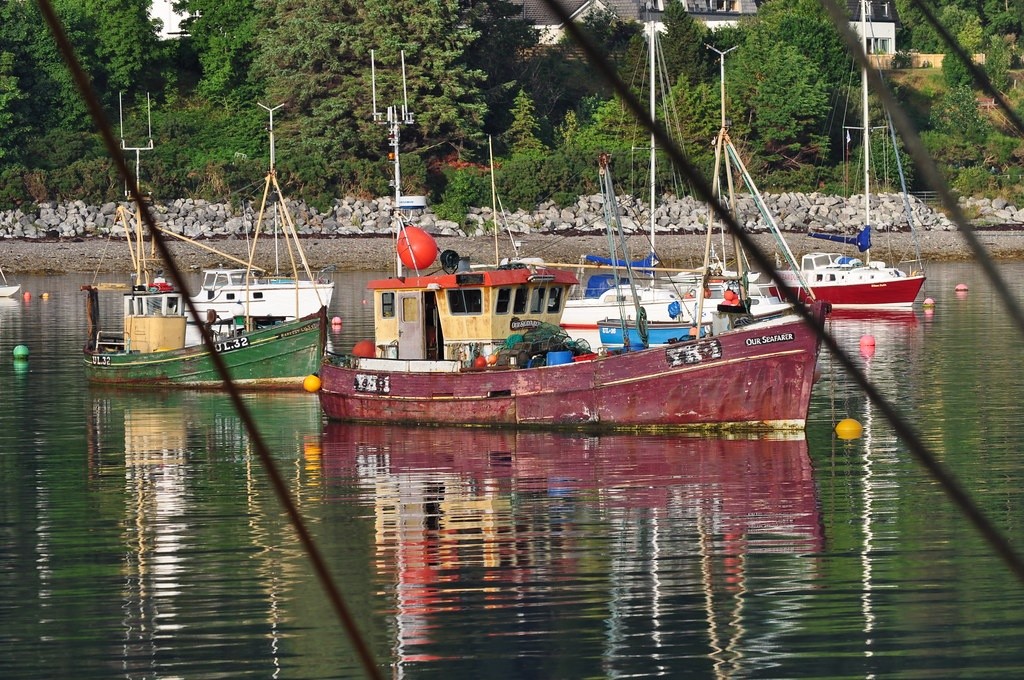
[456,1,929,350]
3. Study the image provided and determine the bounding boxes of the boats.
[81,90,329,386]
[304,43,832,431]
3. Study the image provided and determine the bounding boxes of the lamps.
[426,282,442,290]
[527,275,556,283]
